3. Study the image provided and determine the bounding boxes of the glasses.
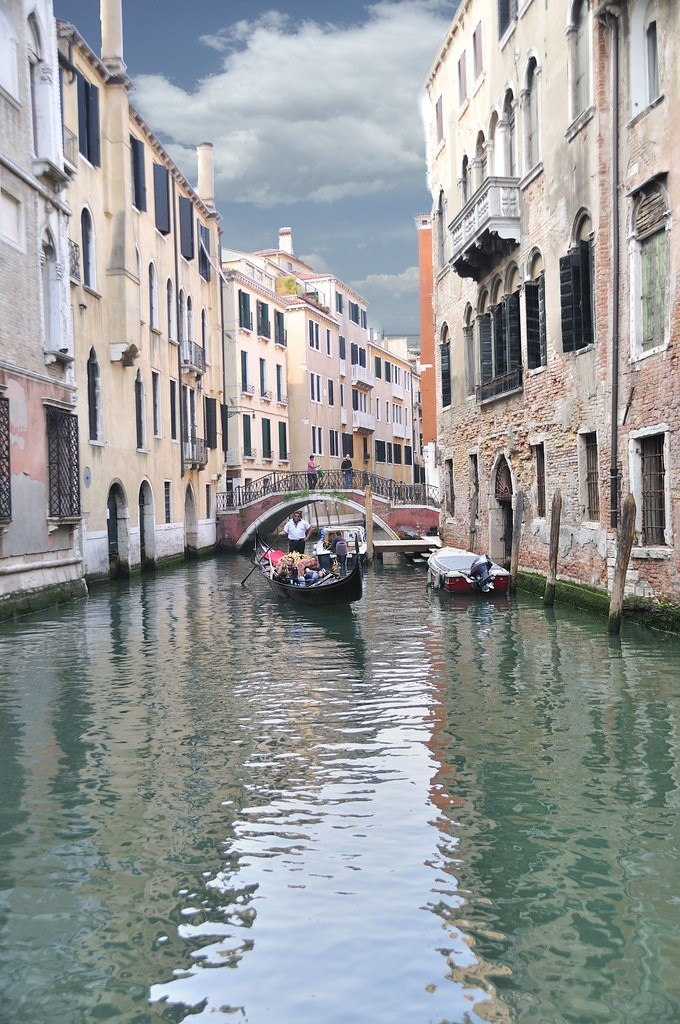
[295,516,299,517]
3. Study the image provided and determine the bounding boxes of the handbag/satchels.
[315,465,324,477]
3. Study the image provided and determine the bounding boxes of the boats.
[250,522,365,603]
[312,524,368,561]
[425,547,509,594]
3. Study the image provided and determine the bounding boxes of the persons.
[330,531,347,563]
[341,453,352,489]
[307,455,321,490]
[277,556,325,582]
[278,512,315,560]
